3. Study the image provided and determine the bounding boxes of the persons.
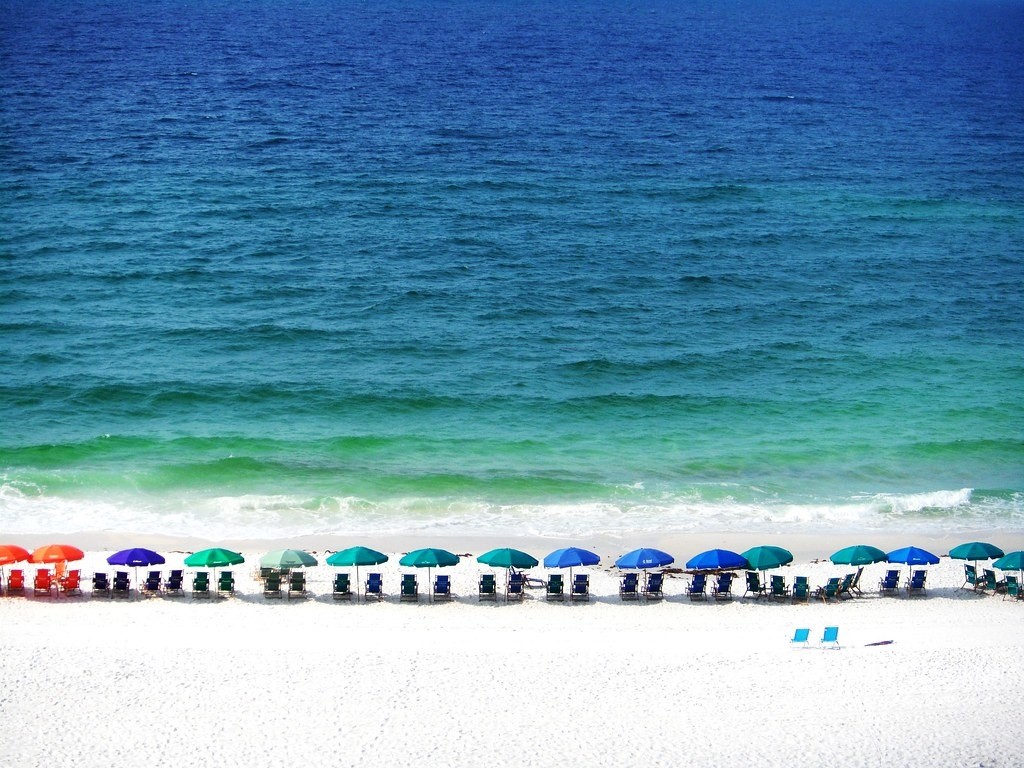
[52,560,66,590]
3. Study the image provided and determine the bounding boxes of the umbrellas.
[476,547,538,601]
[107,548,166,602]
[398,547,460,603]
[829,545,886,588]
[27,543,84,578]
[740,545,793,581]
[0,544,29,566]
[183,548,246,595]
[992,550,1024,584]
[543,546,601,600]
[259,548,319,589]
[326,545,388,603]
[685,547,749,580]
[948,542,1004,569]
[885,546,941,579]
[614,547,675,587]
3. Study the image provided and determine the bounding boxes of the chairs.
[790,628,810,648]
[400,574,419,602]
[819,627,840,649]
[506,573,590,602]
[904,570,927,599]
[878,570,901,598]
[961,564,1024,602]
[363,572,384,602]
[332,573,353,601]
[259,566,307,601]
[743,567,864,606]
[620,571,664,601]
[6,569,235,597]
[685,572,733,603]
[433,574,451,601]
[478,573,496,602]
